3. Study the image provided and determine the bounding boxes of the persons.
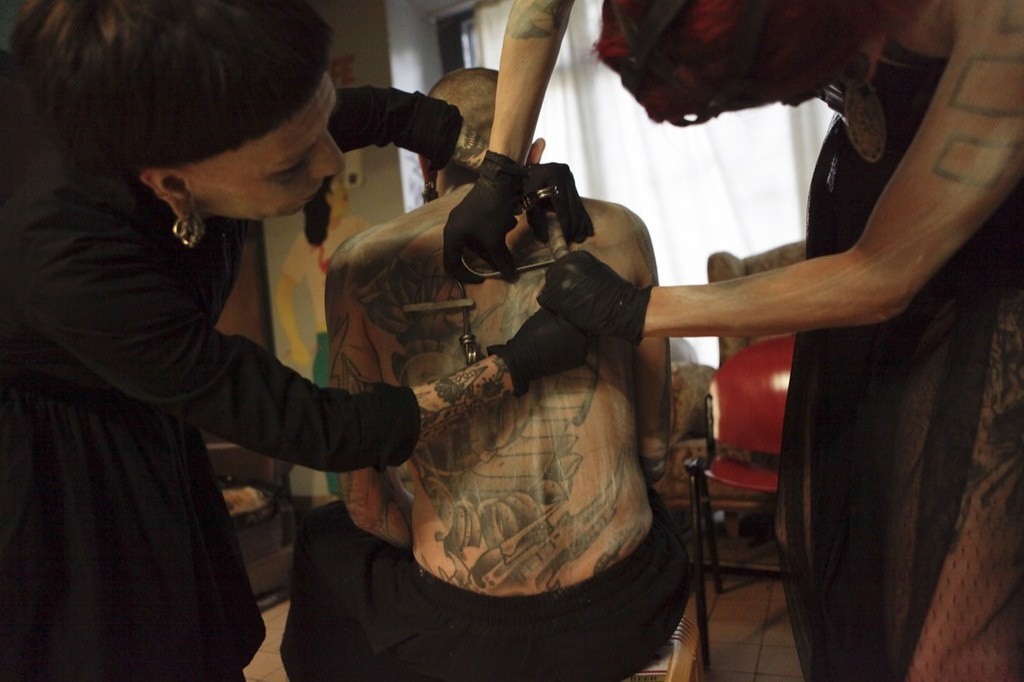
[278,66,687,682]
[0,0,544,682]
[442,0,1024,682]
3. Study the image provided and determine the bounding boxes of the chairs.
[684,335,795,667]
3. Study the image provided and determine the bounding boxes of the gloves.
[486,307,589,397]
[443,150,523,284]
[535,249,654,346]
[523,162,595,244]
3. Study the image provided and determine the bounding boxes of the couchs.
[653,237,806,541]
